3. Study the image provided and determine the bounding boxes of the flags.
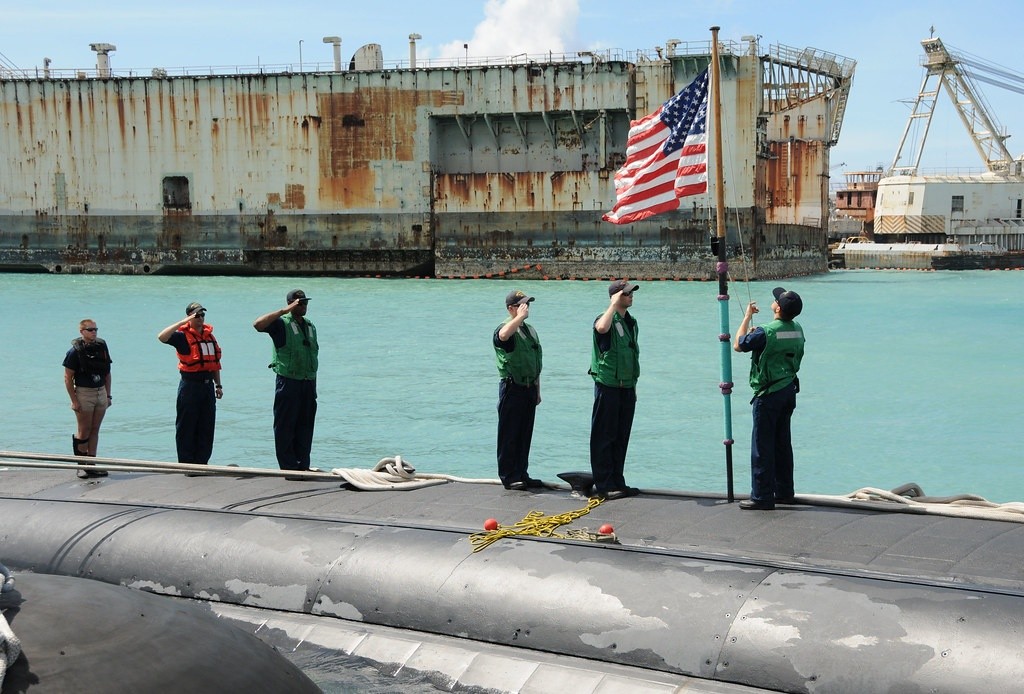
[601,63,712,225]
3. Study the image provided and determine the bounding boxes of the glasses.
[622,292,632,296]
[195,312,205,318]
[298,300,308,305]
[775,298,779,305]
[82,328,99,332]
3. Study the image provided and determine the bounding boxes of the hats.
[506,290,535,306]
[772,287,803,317]
[286,289,312,301]
[186,302,207,314]
[609,280,639,296]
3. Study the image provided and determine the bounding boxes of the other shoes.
[76,469,88,477]
[285,475,306,479]
[86,470,108,476]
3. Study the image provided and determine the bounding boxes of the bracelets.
[216,385,222,389]
[107,396,112,399]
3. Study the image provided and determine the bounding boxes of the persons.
[493,292,542,490]
[158,302,223,477]
[588,281,640,500]
[62,320,113,478]
[734,288,805,510]
[253,289,320,480]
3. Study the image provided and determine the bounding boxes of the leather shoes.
[505,481,523,489]
[621,486,641,496]
[739,499,775,510]
[774,493,794,503]
[526,478,542,486]
[592,485,622,499]
